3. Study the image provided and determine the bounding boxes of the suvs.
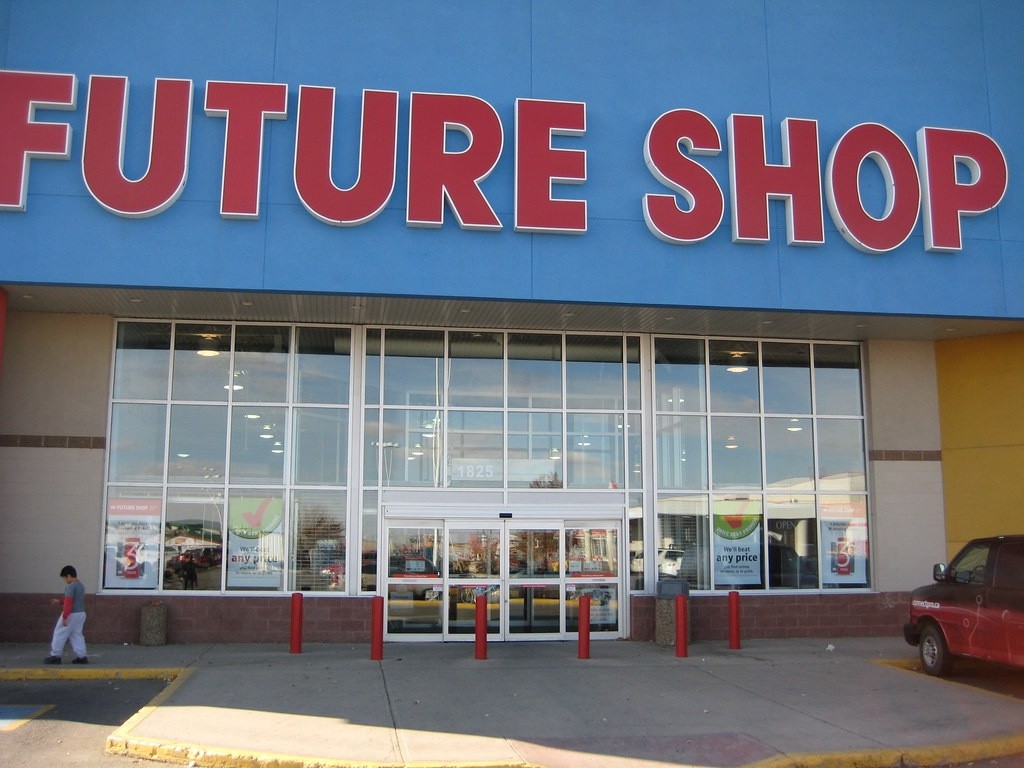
[390,556,441,602]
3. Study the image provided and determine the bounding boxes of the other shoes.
[72,656,88,664]
[43,656,62,665]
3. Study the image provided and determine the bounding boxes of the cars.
[739,544,818,589]
[137,543,180,566]
[903,534,1024,677]
[631,548,686,573]
[320,558,405,592]
[178,548,212,565]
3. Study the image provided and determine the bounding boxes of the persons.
[184,553,199,590]
[44,566,88,664]
[570,586,582,600]
[599,585,611,631]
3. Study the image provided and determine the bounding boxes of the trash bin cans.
[140,605,168,646]
[654,579,691,646]
